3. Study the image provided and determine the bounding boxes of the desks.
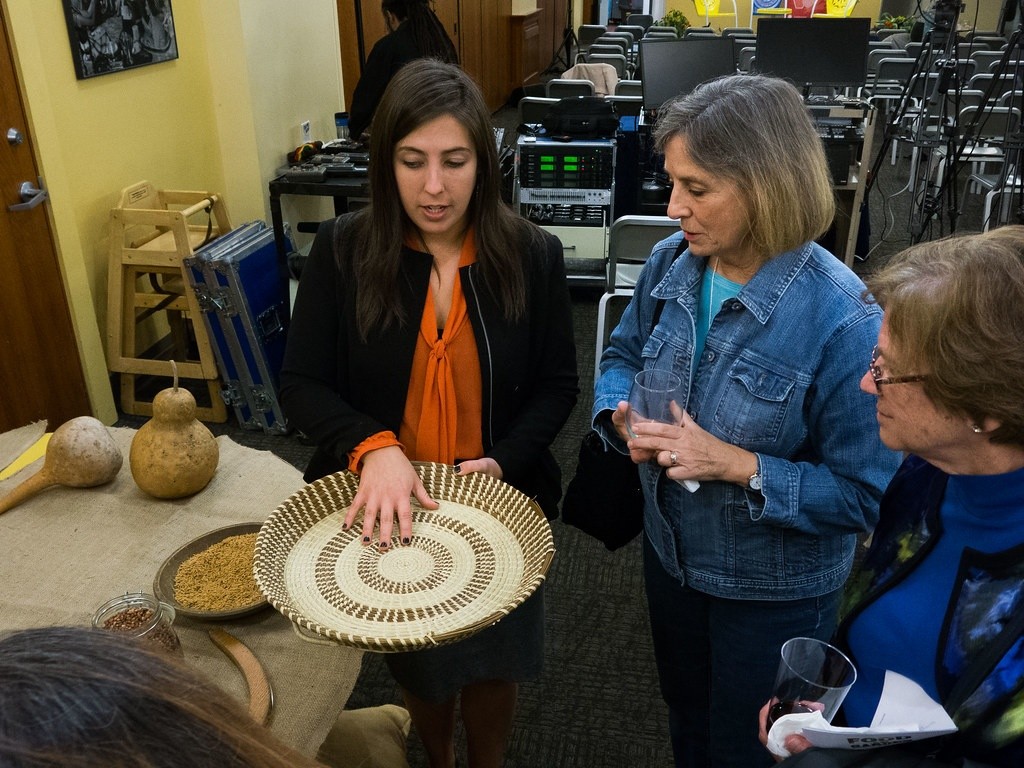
[268,173,379,278]
[0,422,365,768]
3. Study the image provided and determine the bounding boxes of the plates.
[154,521,272,619]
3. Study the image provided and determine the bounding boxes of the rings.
[671,452,677,465]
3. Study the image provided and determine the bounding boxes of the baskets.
[252,461,554,653]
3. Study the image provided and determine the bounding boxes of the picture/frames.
[62,0,179,79]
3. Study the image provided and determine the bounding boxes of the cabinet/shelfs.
[803,98,877,271]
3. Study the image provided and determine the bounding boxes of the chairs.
[513,15,1024,390]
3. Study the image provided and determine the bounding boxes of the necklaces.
[707,257,720,330]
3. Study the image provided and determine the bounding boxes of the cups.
[626,368,684,438]
[766,636,857,739]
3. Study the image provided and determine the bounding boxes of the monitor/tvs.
[640,36,736,110]
[755,18,872,99]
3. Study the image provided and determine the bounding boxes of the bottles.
[92,593,184,660]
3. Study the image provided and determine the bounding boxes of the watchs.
[747,460,762,492]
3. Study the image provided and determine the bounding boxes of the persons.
[290,59,578,768]
[345,0,461,140]
[0,628,328,768]
[757,225,1024,768]
[588,77,910,768]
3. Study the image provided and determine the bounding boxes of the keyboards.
[812,124,865,141]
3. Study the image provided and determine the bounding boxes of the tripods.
[861,5,1024,245]
[546,0,587,77]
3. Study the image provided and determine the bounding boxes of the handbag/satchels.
[561,433,645,550]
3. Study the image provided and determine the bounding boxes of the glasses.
[869,344,932,397]
